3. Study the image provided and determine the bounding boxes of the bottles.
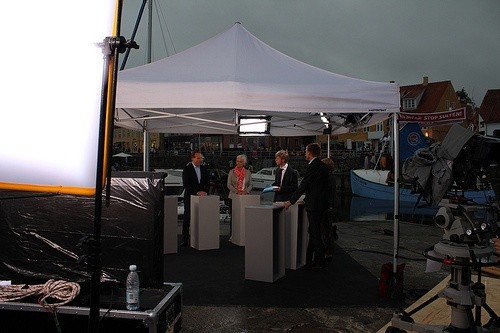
[126,265,140,311]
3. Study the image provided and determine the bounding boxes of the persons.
[492,238,500,254]
[226,153,254,241]
[271,142,334,263]
[164,142,372,169]
[179,152,211,247]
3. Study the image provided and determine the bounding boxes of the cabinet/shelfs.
[190,195,220,250]
[245,205,286,283]
[231,194,260,246]
[277,202,306,283]
[164,196,177,254]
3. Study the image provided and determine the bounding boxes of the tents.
[115,21,401,272]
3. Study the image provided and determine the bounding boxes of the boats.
[250,166,303,191]
[349,135,500,205]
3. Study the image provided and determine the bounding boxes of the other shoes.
[304,261,323,271]
[179,241,188,247]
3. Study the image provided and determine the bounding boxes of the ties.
[278,169,283,186]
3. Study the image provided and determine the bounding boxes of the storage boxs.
[0,282,183,333]
